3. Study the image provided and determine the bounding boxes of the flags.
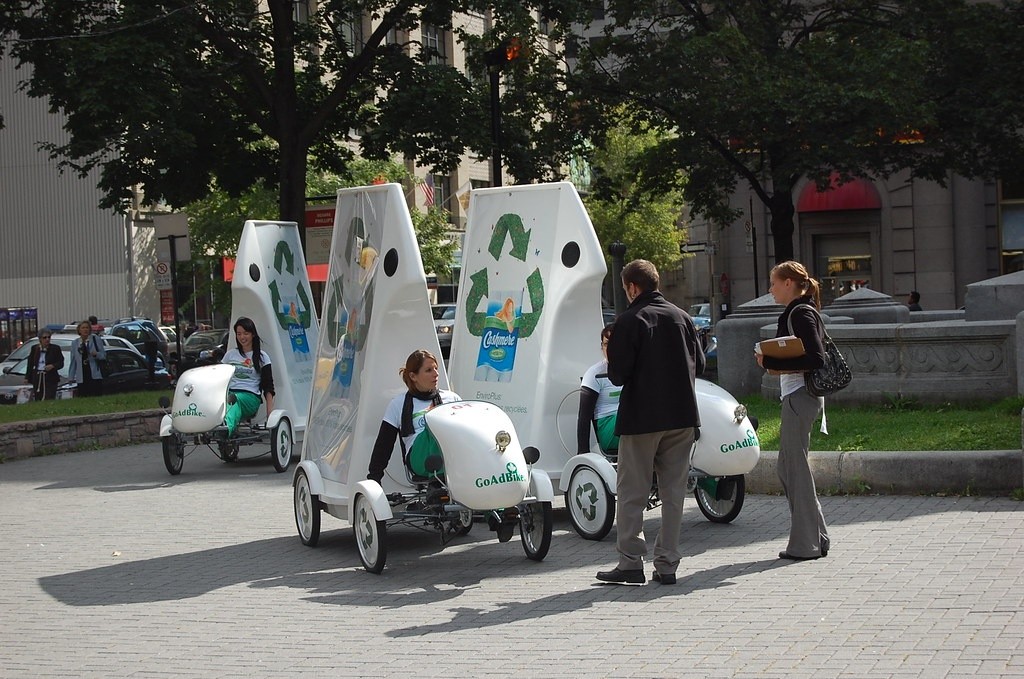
[456,182,473,218]
[419,174,434,206]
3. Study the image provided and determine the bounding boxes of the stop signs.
[720,273,728,296]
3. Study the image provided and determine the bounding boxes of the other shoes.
[426,483,449,503]
[497,505,519,543]
[779,548,828,560]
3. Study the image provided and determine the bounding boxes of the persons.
[754,261,831,559]
[68,320,106,398]
[908,291,922,311]
[218,317,275,440]
[24,327,64,402]
[367,349,519,543]
[595,259,706,584]
[578,323,624,455]
[181,322,205,339]
[89,316,104,331]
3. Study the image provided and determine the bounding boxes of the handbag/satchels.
[787,304,854,396]
[94,335,113,378]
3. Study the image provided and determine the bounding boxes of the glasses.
[602,342,608,347]
[39,336,51,339]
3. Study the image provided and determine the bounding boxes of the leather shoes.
[596,567,645,584]
[653,569,676,584]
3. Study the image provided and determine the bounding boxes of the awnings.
[796,169,882,212]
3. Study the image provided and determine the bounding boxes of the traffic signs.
[680,243,707,254]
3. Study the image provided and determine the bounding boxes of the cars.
[0,316,229,406]
[428,303,457,349]
[688,303,718,356]
